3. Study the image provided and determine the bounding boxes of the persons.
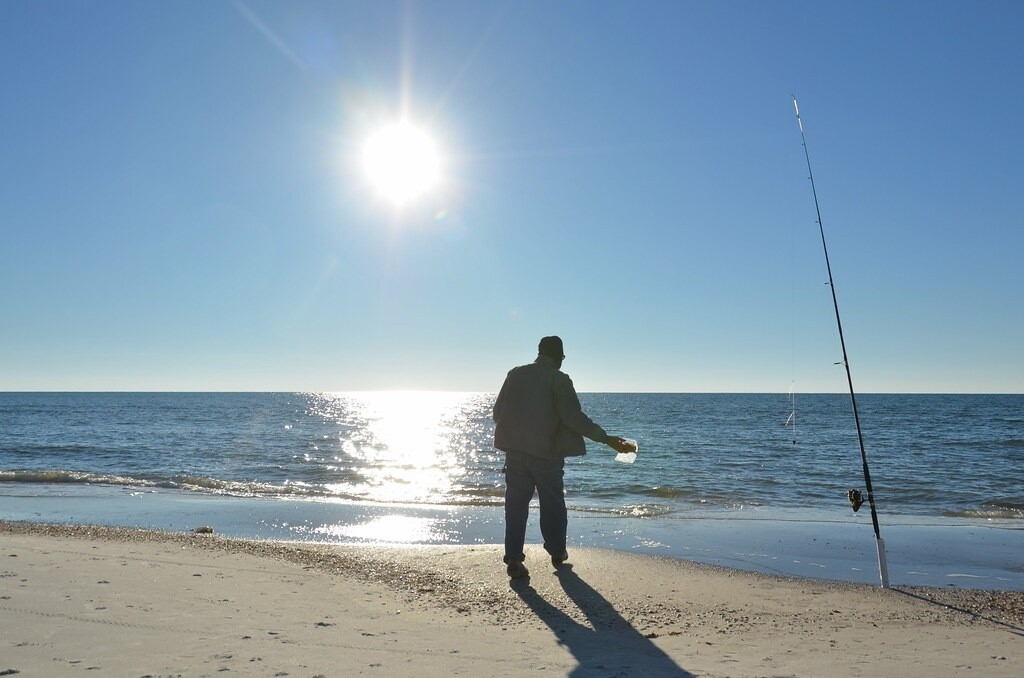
[492,335,626,578]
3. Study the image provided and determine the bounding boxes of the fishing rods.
[790,94,892,589]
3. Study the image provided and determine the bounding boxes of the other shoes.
[552,551,568,565]
[507,562,529,578]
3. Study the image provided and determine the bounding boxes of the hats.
[538,336,563,356]
